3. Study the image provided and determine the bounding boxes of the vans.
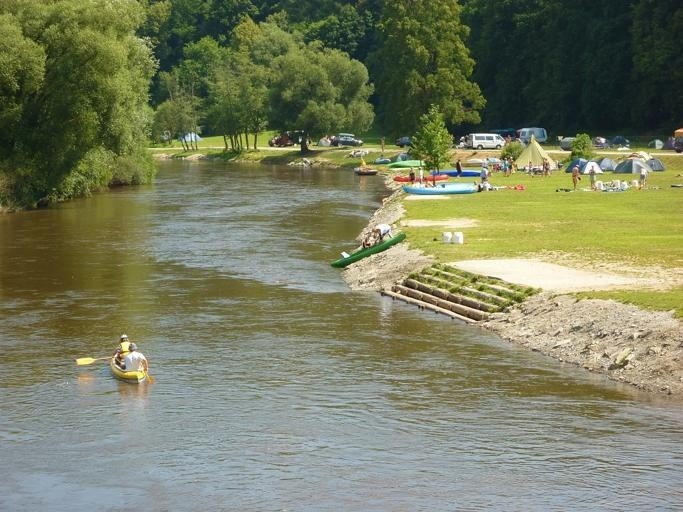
[520,128,548,144]
[468,133,506,150]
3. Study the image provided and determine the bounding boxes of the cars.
[332,133,363,146]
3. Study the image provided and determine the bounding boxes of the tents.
[634,151,652,161]
[602,158,614,170]
[566,158,587,172]
[581,163,603,172]
[648,138,666,150]
[614,158,652,172]
[649,159,664,170]
[512,134,556,170]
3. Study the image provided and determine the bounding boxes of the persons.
[573,165,579,190]
[362,238,371,248]
[479,156,551,190]
[371,224,393,243]
[590,166,597,188]
[380,136,385,152]
[639,167,648,191]
[115,335,132,368]
[418,167,424,183]
[455,159,462,177]
[121,343,150,372]
[410,168,416,184]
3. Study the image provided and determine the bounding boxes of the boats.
[354,158,501,194]
[330,232,406,268]
[111,352,147,384]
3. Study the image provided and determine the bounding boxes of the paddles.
[145,371,154,382]
[76,357,112,365]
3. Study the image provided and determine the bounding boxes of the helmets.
[121,334,128,339]
[128,343,138,351]
